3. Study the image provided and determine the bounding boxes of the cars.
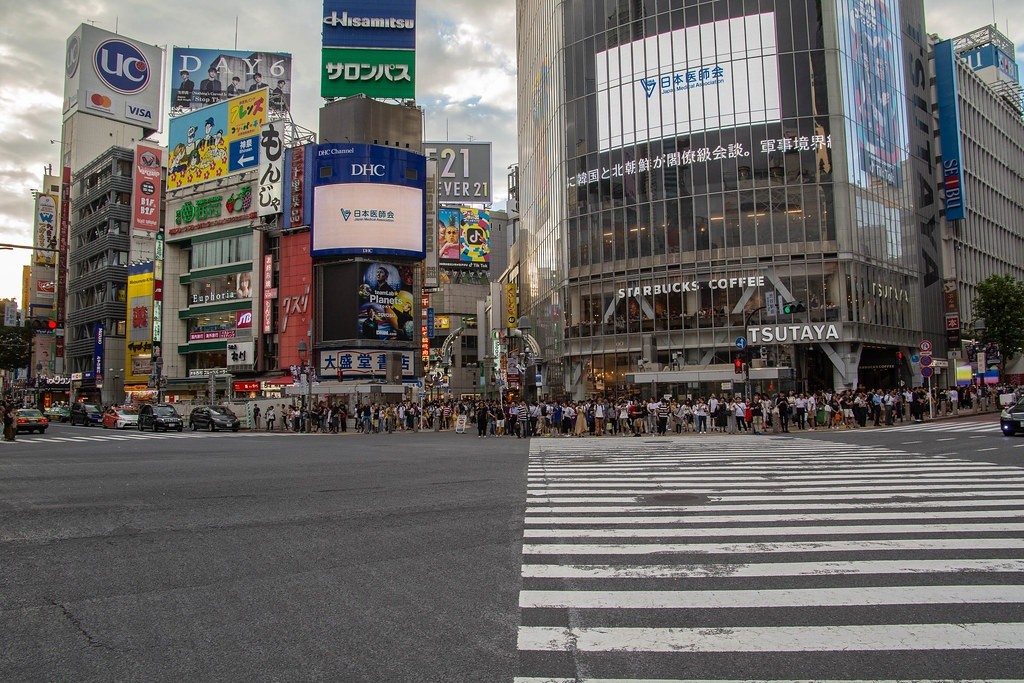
[189,405,240,433]
[101,406,140,430]
[43,405,71,424]
[70,401,103,426]
[14,409,49,434]
[1000,395,1024,437]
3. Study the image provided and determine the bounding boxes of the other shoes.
[699,431,702,434]
[808,428,814,432]
[622,433,625,436]
[633,433,638,436]
[704,431,707,433]
[637,434,641,437]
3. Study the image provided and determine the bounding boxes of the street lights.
[467,362,477,423]
[35,360,44,410]
[297,337,308,431]
[156,356,163,404]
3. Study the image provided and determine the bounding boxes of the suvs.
[139,404,183,433]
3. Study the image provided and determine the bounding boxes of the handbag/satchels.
[835,413,841,421]
[509,413,517,425]
[396,418,400,427]
[646,407,654,414]
[606,422,612,431]
[881,395,890,403]
[636,408,644,418]
[671,415,674,421]
[724,408,732,416]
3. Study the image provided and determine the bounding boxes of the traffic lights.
[24,318,57,332]
[735,358,742,374]
[783,303,806,315]
[896,351,903,367]
[337,370,343,383]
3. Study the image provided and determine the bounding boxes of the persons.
[238,272,252,298]
[0,396,37,441]
[51,400,122,412]
[173,67,289,112]
[362,268,413,341]
[439,219,459,258]
[253,383,1024,440]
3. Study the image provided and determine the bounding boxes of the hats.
[331,402,336,405]
[554,400,560,403]
[711,394,715,398]
